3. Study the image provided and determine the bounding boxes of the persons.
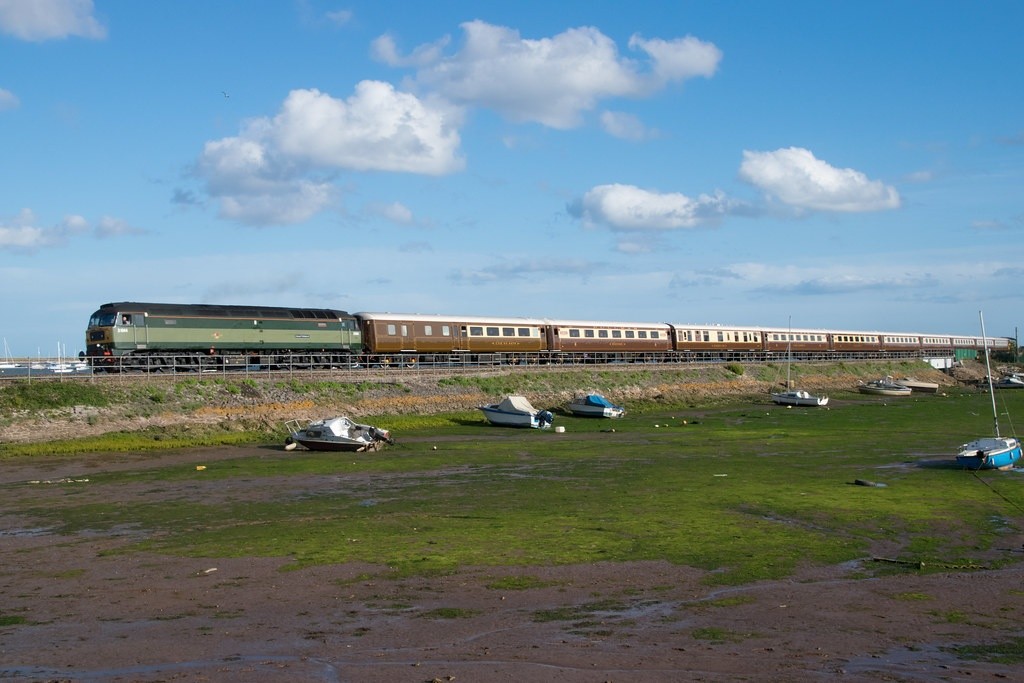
[122,316,129,325]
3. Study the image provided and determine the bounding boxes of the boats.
[478,396,554,430]
[896,377,939,389]
[285,415,393,452]
[858,377,912,395]
[997,377,1024,387]
[557,394,627,419]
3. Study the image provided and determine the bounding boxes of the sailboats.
[769,316,829,407]
[0,336,91,373]
[956,311,1022,468]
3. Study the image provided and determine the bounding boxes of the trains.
[84,303,1018,371]
